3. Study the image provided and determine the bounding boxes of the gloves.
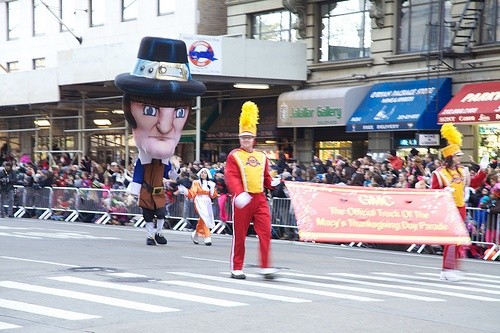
[479,154,489,170]
[271,178,281,187]
[444,187,455,193]
[234,193,253,210]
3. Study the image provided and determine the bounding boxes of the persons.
[116,36,205,246]
[188,167,217,245]
[0,155,145,227]
[460,157,500,260]
[433,144,489,280]
[164,153,257,237]
[224,101,289,278]
[268,149,445,254]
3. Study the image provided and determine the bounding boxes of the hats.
[6,163,10,167]
[440,123,464,157]
[387,149,397,158]
[238,101,259,137]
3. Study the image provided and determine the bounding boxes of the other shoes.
[281,237,290,240]
[264,273,274,279]
[191,234,198,244]
[441,270,461,282]
[295,237,299,241]
[9,215,14,218]
[231,272,245,279]
[205,243,211,245]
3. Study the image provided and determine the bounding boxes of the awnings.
[277,84,373,128]
[346,79,452,132]
[438,82,500,123]
